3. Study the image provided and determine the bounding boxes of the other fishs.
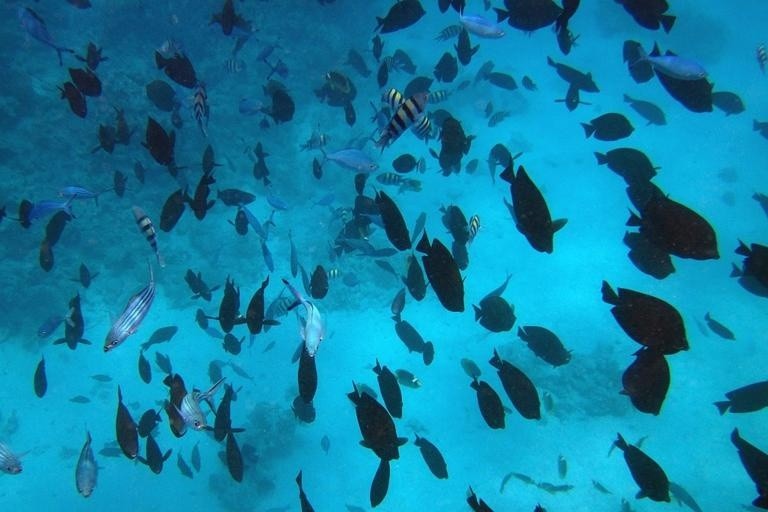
[0,0,768,512]
